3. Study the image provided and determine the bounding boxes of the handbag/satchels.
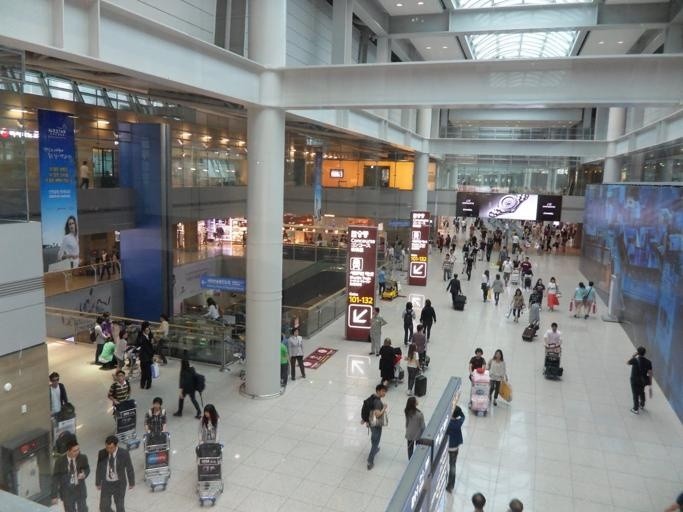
[544,351,560,367]
[583,296,588,301]
[545,366,563,377]
[497,380,514,402]
[555,290,562,299]
[643,375,652,386]
[569,301,573,312]
[367,407,389,428]
[150,360,160,378]
[592,303,597,314]
[529,320,539,330]
[544,343,562,356]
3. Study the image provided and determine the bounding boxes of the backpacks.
[361,395,377,422]
[184,367,205,392]
[404,310,413,326]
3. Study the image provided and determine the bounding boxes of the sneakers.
[630,402,643,416]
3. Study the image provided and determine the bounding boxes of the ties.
[68,458,75,486]
[108,453,115,480]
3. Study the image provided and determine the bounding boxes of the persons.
[507,499,525,511]
[363,304,385,356]
[78,160,93,186]
[374,266,390,294]
[420,298,435,342]
[378,335,397,388]
[405,397,425,460]
[89,242,121,282]
[401,300,415,345]
[359,387,388,468]
[53,212,80,267]
[198,217,410,261]
[444,407,465,493]
[662,492,682,512]
[471,492,487,512]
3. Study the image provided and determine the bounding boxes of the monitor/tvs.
[330,169,344,178]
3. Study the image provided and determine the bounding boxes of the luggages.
[414,374,427,397]
[521,327,536,341]
[453,293,466,311]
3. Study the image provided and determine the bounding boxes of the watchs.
[487,193,528,217]
